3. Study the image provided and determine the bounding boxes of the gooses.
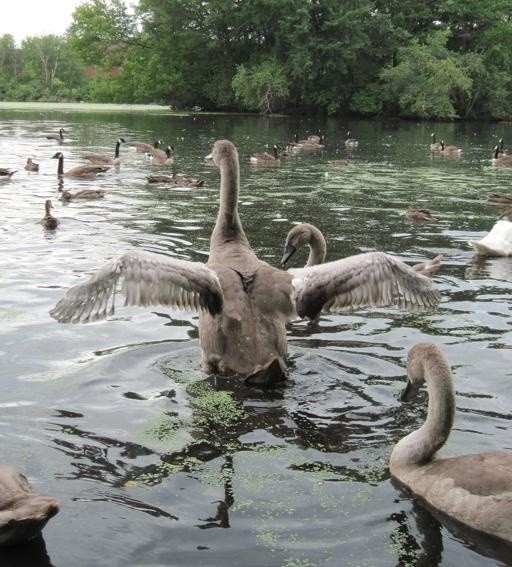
[490,136,512,167]
[248,127,361,165]
[468,204,512,260]
[388,342,511,543]
[47,138,443,387]
[279,222,443,279]
[45,129,69,146]
[0,137,205,201]
[487,192,512,204]
[0,464,60,546]
[438,140,462,157]
[34,200,60,232]
[405,201,439,223]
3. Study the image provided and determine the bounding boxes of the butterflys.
[430,132,449,151]
